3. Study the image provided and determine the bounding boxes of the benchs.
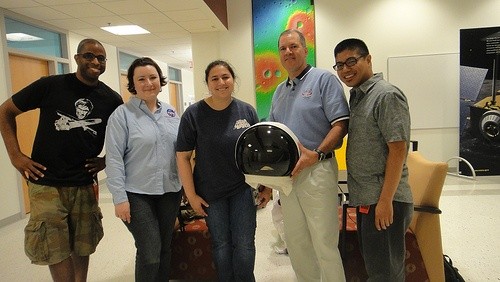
[338,152,445,282]
[173,196,216,282]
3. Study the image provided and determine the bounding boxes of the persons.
[269,30,349,282]
[106,57,184,282]
[0,38,117,282]
[176,61,270,282]
[335,39,413,282]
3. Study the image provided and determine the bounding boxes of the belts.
[324,151,337,161]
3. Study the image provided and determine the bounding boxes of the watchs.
[315,150,325,160]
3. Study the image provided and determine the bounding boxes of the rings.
[123,218,126,220]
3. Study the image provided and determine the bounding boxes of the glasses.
[332,52,365,73]
[74,50,108,64]
